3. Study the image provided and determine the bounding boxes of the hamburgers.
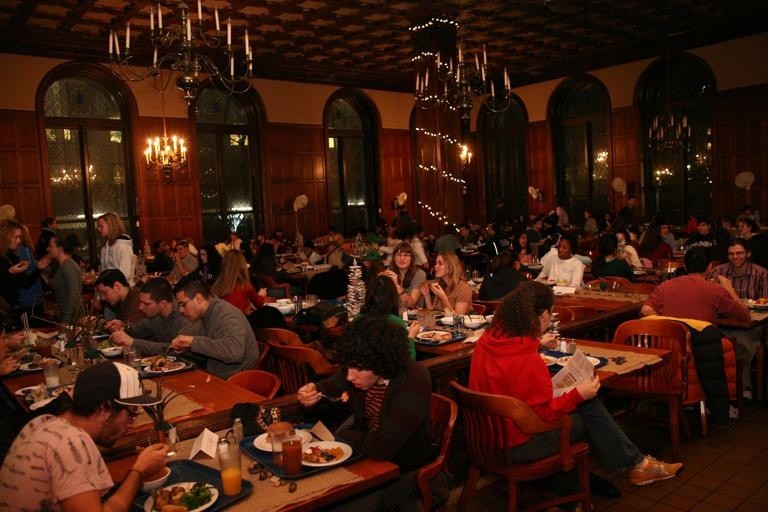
[266,423,295,441]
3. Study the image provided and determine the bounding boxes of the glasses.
[111,400,140,425]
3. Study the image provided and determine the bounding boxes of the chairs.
[450,380,598,509]
[607,319,688,462]
[642,313,711,435]
[0,225,767,402]
[405,390,458,509]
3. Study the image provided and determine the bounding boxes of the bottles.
[217,437,229,467]
[80,264,97,286]
[233,417,244,443]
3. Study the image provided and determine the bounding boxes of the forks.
[320,395,340,402]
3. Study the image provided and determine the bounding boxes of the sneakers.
[627,454,688,488]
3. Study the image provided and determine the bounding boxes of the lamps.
[460,143,471,170]
[641,0,697,154]
[105,0,255,104]
[144,68,188,183]
[415,0,514,121]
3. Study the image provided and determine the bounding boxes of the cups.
[270,436,290,468]
[218,441,243,498]
[135,248,161,284]
[672,240,711,257]
[559,224,575,232]
[467,271,482,285]
[281,436,302,476]
[527,258,540,266]
[458,242,478,254]
[278,256,319,274]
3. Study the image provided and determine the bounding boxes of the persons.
[1,361,169,509]
[296,312,435,512]
[706,237,766,420]
[0,201,757,361]
[465,279,685,489]
[169,270,260,381]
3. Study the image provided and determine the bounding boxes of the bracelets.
[124,469,144,479]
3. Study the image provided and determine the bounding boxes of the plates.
[252,429,312,453]
[142,482,218,512]
[739,295,767,309]
[632,270,646,275]
[14,312,187,416]
[399,298,495,346]
[302,440,352,468]
[538,311,600,369]
[263,292,319,316]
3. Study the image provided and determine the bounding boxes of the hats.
[73,361,164,406]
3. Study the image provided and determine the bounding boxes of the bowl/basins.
[138,466,172,493]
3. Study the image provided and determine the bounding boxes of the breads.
[155,486,188,512]
[421,330,449,342]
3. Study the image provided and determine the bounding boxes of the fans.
[0,203,16,219]
[612,177,628,196]
[528,186,544,200]
[734,170,754,191]
[391,192,408,208]
[292,193,309,212]
